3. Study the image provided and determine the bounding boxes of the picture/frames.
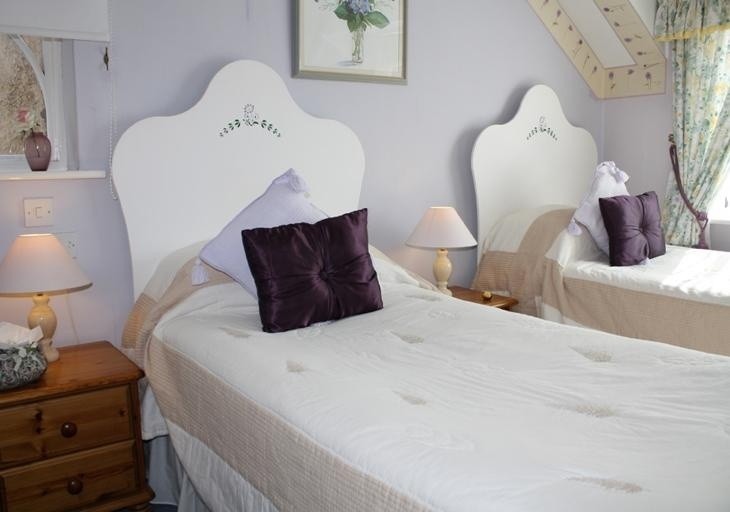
[291,0,408,85]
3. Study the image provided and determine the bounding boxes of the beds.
[469,84,729,356]
[111,58,730,512]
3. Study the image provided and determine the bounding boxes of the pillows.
[598,191,666,266]
[197,167,331,299]
[566,160,631,257]
[240,208,383,333]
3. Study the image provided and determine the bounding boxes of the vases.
[24,133,52,171]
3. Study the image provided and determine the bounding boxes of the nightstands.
[0,340,156,512]
[448,285,520,312]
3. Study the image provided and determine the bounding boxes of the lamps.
[0,234,93,363]
[405,206,478,297]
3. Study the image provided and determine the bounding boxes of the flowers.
[347,0,369,15]
[15,109,44,158]
